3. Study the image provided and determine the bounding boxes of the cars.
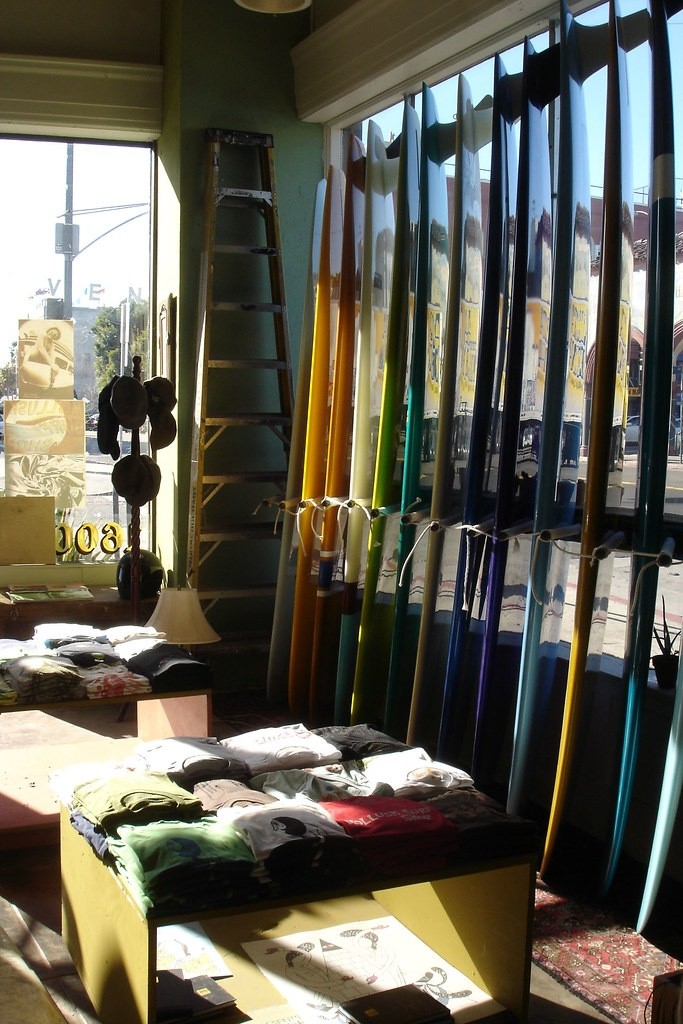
[85,413,102,431]
[625,414,640,445]
[0,413,4,441]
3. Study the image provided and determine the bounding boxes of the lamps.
[147,583,221,658]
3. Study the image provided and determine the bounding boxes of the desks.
[0,688,244,851]
[0,585,159,638]
[58,798,536,1024]
